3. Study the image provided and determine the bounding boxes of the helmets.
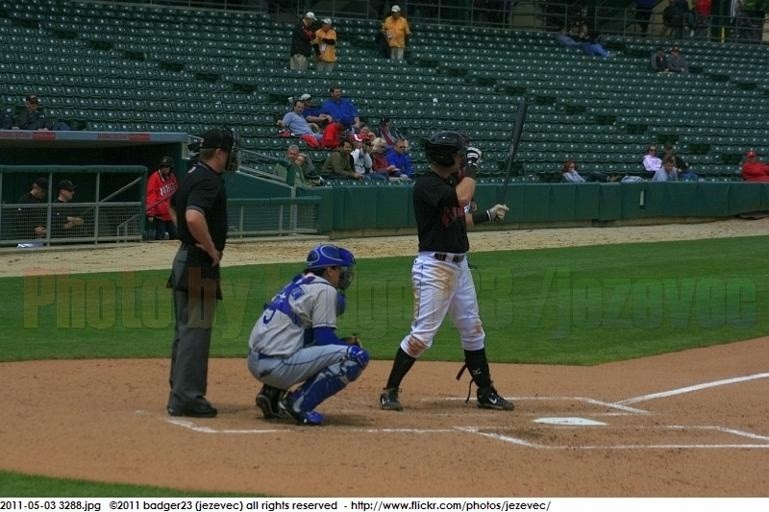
[306,244,356,272]
[424,131,467,169]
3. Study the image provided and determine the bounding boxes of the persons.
[384,5,413,61]
[16,93,72,131]
[41,178,85,245]
[289,11,317,72]
[247,244,368,425]
[559,159,622,183]
[165,129,238,418]
[16,177,46,247]
[648,46,668,72]
[581,37,617,58]
[0,109,21,131]
[640,145,663,171]
[652,156,678,182]
[741,151,769,182]
[454,154,478,213]
[659,142,688,167]
[310,18,337,73]
[146,155,179,239]
[377,129,515,410]
[554,29,577,46]
[278,86,417,180]
[633,1,769,42]
[667,46,689,72]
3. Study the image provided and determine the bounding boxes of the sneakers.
[476,387,516,411]
[279,395,325,426]
[255,393,282,421]
[168,401,219,418]
[379,389,403,411]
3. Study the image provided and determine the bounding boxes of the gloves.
[465,146,483,167]
[487,203,509,221]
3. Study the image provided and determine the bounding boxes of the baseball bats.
[495,96,529,223]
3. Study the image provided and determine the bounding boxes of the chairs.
[0,0,768,183]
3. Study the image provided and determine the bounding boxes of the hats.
[321,18,332,24]
[747,152,755,157]
[160,155,174,168]
[27,95,41,102]
[391,5,401,12]
[199,129,235,152]
[299,93,311,100]
[59,180,77,190]
[306,12,317,20]
[37,178,48,192]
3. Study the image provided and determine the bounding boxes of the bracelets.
[472,210,492,226]
[463,167,478,181]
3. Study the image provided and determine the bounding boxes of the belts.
[435,253,467,263]
